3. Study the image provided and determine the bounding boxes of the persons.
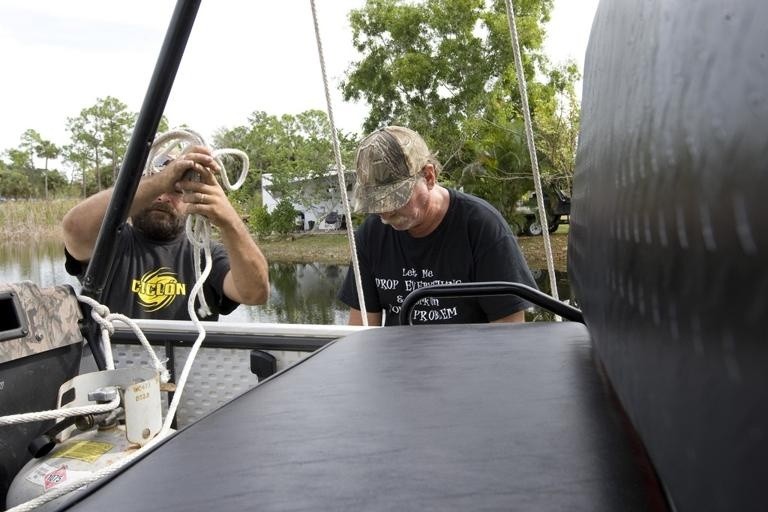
[60,144,271,322]
[337,126,540,326]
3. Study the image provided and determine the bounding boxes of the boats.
[0,0,768,511]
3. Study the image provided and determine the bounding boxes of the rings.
[200,193,209,203]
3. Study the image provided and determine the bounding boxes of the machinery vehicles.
[514,175,573,241]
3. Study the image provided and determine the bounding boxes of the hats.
[353,126,430,214]
